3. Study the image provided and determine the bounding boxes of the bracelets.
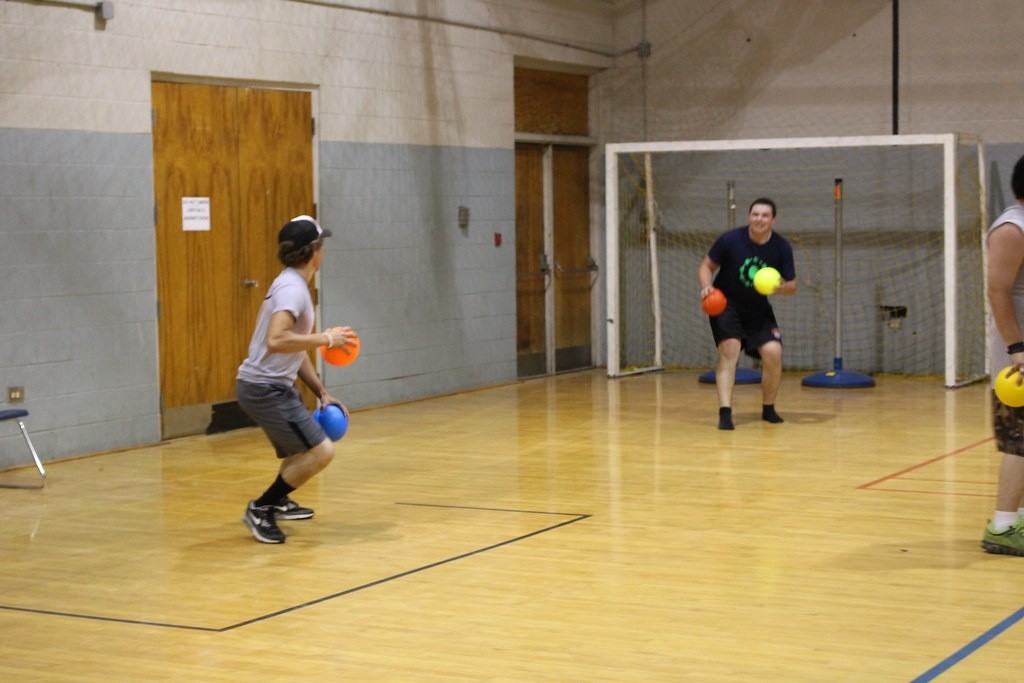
[322,333,334,350]
[1006,342,1024,355]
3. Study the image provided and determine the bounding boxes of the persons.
[699,197,797,432]
[235,214,357,544]
[979,155,1024,558]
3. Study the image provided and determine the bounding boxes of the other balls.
[995,366,1024,408]
[703,288,727,316]
[314,403,348,443]
[753,267,783,294]
[319,326,360,365]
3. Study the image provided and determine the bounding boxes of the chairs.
[0,410,48,490]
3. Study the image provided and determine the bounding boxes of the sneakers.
[273,496,314,520]
[980,519,1024,556]
[244,500,285,543]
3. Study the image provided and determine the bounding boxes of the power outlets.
[7,386,24,402]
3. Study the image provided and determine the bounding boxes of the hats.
[278,215,332,253]
